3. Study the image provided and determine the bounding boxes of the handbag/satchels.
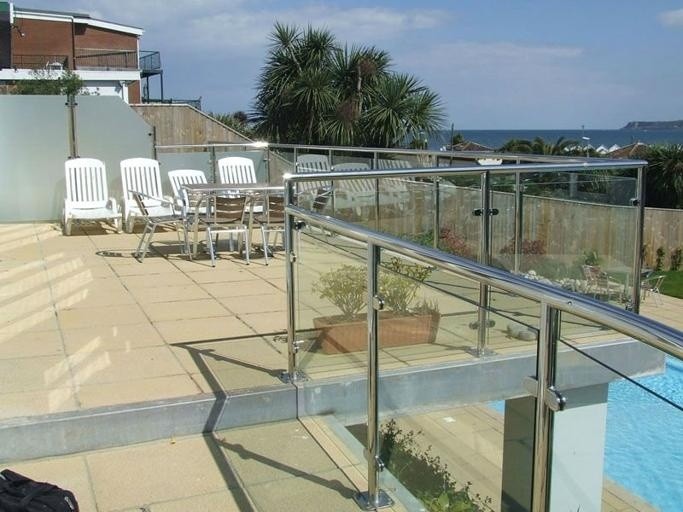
[1,470,80,512]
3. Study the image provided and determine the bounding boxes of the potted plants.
[311,257,441,355]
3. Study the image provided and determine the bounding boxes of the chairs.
[524,265,667,308]
[297,154,416,217]
[63,156,334,268]
